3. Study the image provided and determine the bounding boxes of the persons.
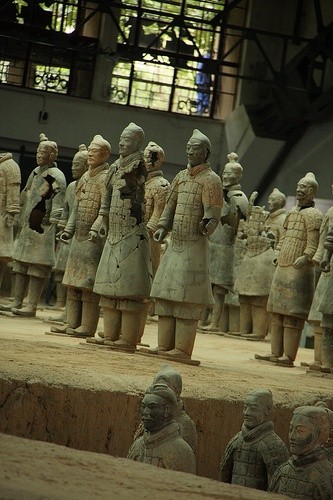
[0,131,67,316]
[53,143,90,311]
[50,134,113,336]
[255,171,323,367]
[194,48,216,117]
[134,370,197,455]
[219,387,289,491]
[267,401,333,500]
[228,186,288,340]
[96,140,171,344]
[300,204,333,368]
[198,153,250,333]
[140,129,225,360]
[126,384,197,475]
[0,152,23,263]
[311,225,332,373]
